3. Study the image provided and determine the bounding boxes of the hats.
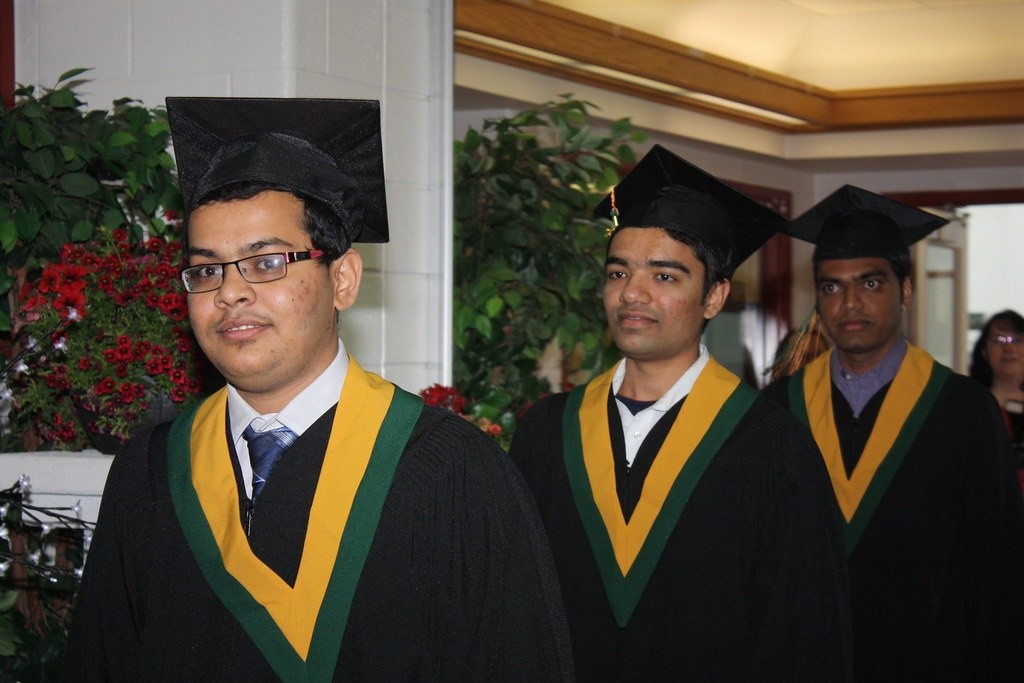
[592,143,791,275]
[165,95,390,245]
[781,184,951,272]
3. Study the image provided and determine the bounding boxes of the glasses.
[177,250,324,293]
[986,332,1024,346]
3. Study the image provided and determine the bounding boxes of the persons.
[45,98,575,683]
[755,183,1024,683]
[769,324,832,383]
[969,310,1024,498]
[506,146,854,683]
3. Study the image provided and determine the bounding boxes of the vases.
[66,376,183,457]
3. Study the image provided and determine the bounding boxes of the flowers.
[422,383,503,438]
[516,380,576,420]
[18,209,216,448]
